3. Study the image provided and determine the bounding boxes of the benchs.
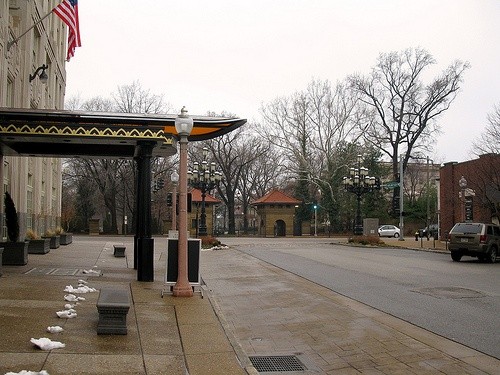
[113,244,127,257]
[95,284,131,337]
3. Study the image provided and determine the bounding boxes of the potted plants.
[0,191,30,266]
[53,226,73,245]
[41,227,61,249]
[25,228,50,255]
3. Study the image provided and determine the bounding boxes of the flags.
[51,0,82,62]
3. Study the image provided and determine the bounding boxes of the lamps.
[39,71,49,84]
[170,169,179,182]
[175,105,193,139]
[186,145,224,192]
[341,152,376,195]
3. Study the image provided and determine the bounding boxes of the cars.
[378,224,401,237]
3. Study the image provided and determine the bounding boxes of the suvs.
[447,221,500,263]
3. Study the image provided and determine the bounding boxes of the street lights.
[171,168,179,231]
[459,174,468,222]
[175,103,195,296]
[341,151,379,236]
[185,145,225,236]
